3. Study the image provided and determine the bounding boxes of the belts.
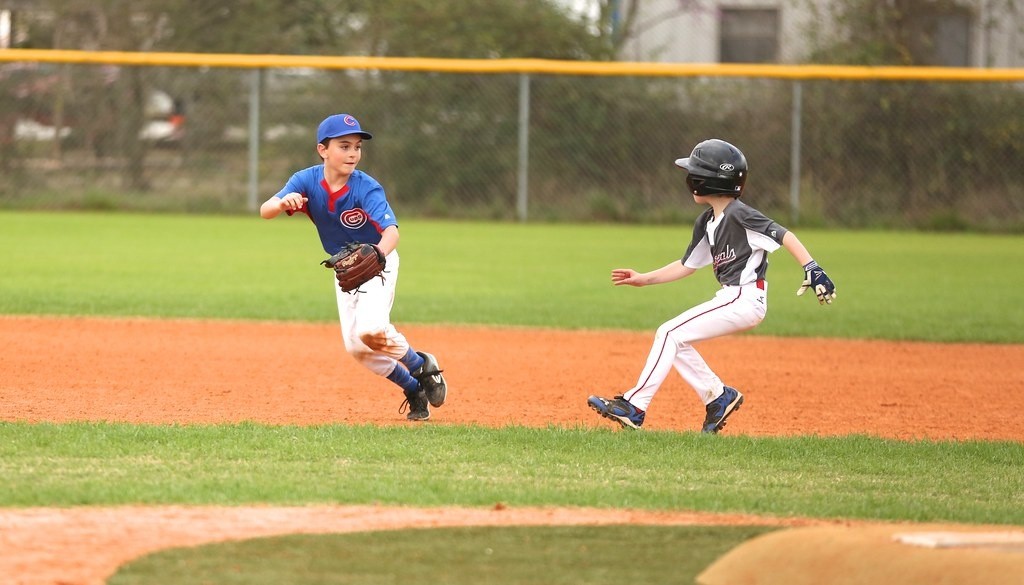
[756,279,765,289]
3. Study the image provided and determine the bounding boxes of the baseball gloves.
[320,241,386,295]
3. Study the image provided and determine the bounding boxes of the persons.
[587,139,837,434]
[260,114,447,421]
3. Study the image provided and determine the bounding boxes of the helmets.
[675,139,747,196]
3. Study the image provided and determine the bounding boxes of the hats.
[317,114,372,143]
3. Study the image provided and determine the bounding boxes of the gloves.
[796,261,837,305]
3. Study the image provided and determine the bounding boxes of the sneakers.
[701,384,743,436]
[588,395,644,430]
[399,381,429,420]
[410,351,447,406]
